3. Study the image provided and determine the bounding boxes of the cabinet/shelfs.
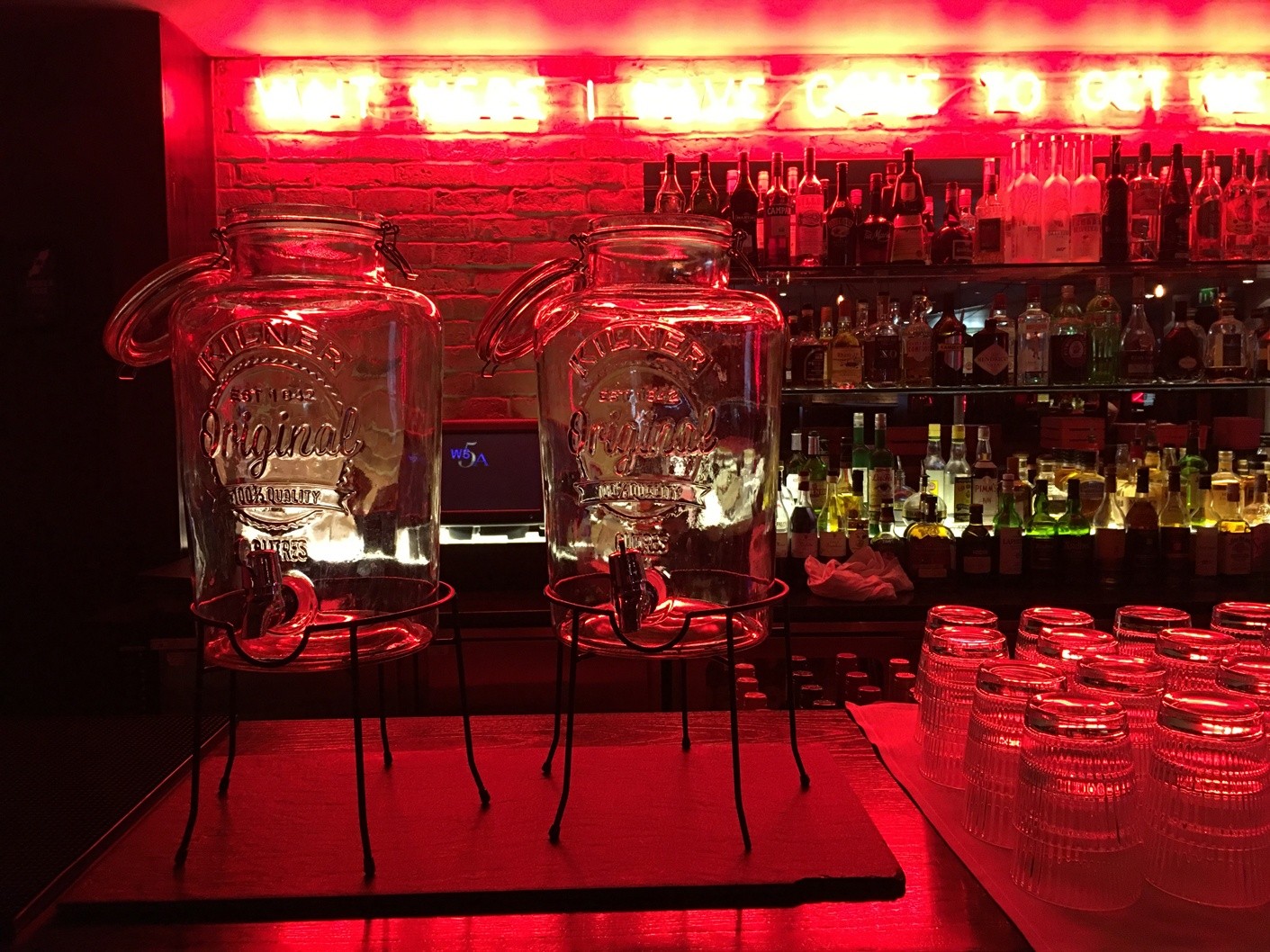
[641,161,1270,622]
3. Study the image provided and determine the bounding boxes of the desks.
[16,710,1030,952]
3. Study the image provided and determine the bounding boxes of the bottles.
[791,655,807,671]
[734,662,756,678]
[771,393,1270,595]
[472,213,789,659]
[835,654,858,693]
[104,204,440,672]
[743,692,767,710]
[837,671,868,708]
[891,673,916,703]
[798,685,823,709]
[812,699,836,709]
[782,275,1270,390]
[856,686,882,705]
[791,670,814,702]
[652,130,1270,281]
[735,677,759,710]
[888,658,910,694]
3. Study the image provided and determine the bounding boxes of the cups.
[913,596,1270,914]
